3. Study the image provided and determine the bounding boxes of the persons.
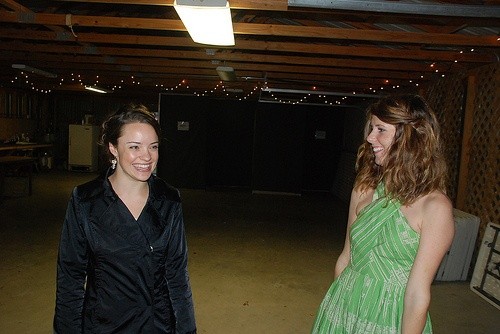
[54,104,199,334]
[310,94,454,334]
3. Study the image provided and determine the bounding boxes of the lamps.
[216,67,236,82]
[173,0,236,46]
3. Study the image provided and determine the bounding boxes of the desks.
[0,144,57,173]
[0,156,38,196]
